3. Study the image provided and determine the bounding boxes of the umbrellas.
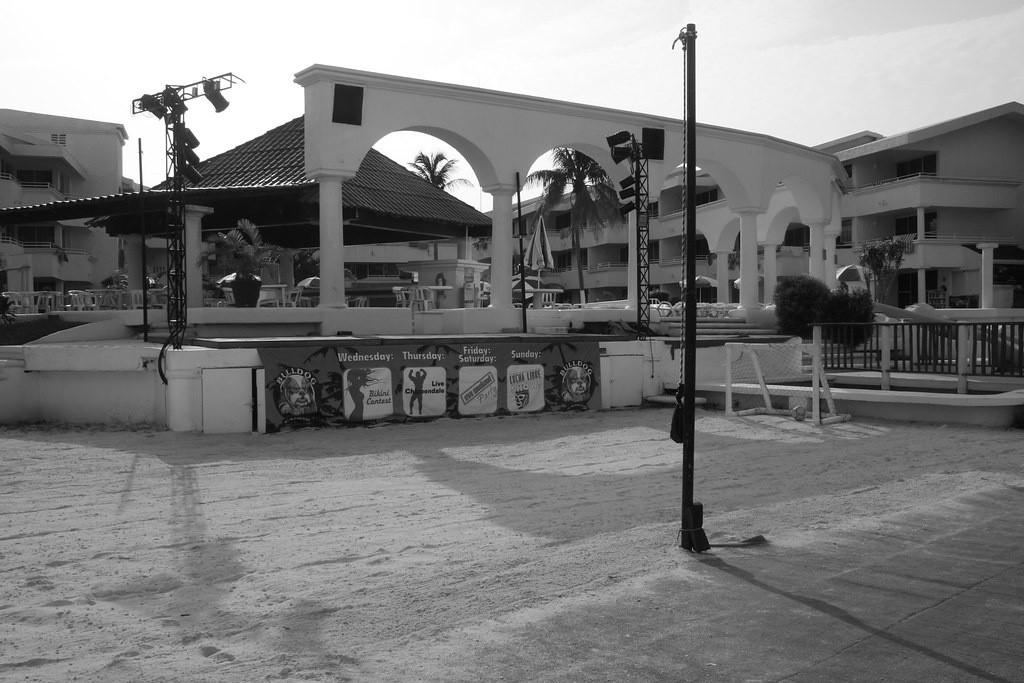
[733,275,764,290]
[298,276,320,288]
[523,215,555,289]
[679,276,718,304]
[217,272,261,285]
[512,273,543,300]
[836,264,878,282]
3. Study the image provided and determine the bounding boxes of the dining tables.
[148,289,168,310]
[513,289,563,309]
[655,303,739,319]
[84,289,123,310]
[401,286,453,309]
[257,284,287,307]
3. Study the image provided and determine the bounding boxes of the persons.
[840,281,848,294]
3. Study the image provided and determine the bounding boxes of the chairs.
[655,300,740,319]
[203,288,236,307]
[511,302,582,309]
[542,292,556,309]
[23,289,129,314]
[0,292,24,314]
[285,287,366,307]
[649,298,661,305]
[128,290,150,310]
[407,286,434,312]
[392,286,410,307]
